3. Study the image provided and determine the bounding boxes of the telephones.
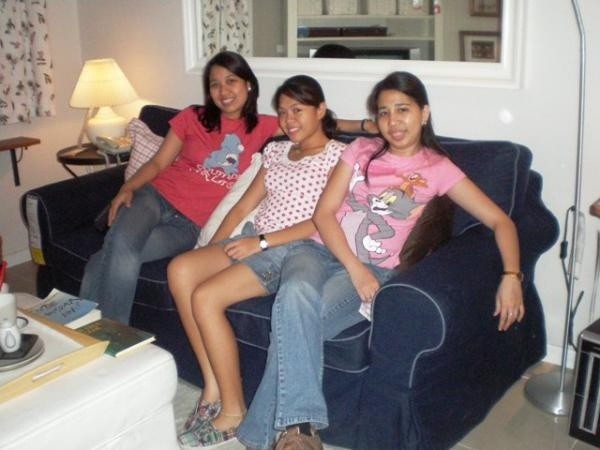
[94,136,133,170]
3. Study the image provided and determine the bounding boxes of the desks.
[56,142,130,177]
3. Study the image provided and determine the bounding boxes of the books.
[26,288,102,330]
[75,317,156,357]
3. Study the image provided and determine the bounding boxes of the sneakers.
[178,421,242,450]
[181,401,222,434]
[274,427,324,450]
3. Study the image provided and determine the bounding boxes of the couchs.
[20,106,560,449]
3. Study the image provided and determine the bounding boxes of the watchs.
[501,270,526,280]
[259,235,268,251]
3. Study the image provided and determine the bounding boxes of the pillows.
[125,116,164,182]
[396,195,451,271]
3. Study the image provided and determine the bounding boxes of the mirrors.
[181,0,526,89]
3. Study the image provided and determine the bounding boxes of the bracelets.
[361,119,372,131]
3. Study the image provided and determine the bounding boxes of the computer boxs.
[567,319,600,449]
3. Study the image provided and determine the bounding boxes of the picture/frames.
[468,1,501,17]
[459,30,500,62]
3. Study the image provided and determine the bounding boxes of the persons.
[168,75,348,450]
[235,71,525,450]
[79,51,379,326]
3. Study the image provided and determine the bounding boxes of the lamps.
[69,58,139,147]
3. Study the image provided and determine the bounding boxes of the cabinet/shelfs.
[0,135,41,187]
[283,0,443,61]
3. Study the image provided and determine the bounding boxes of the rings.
[507,312,516,318]
